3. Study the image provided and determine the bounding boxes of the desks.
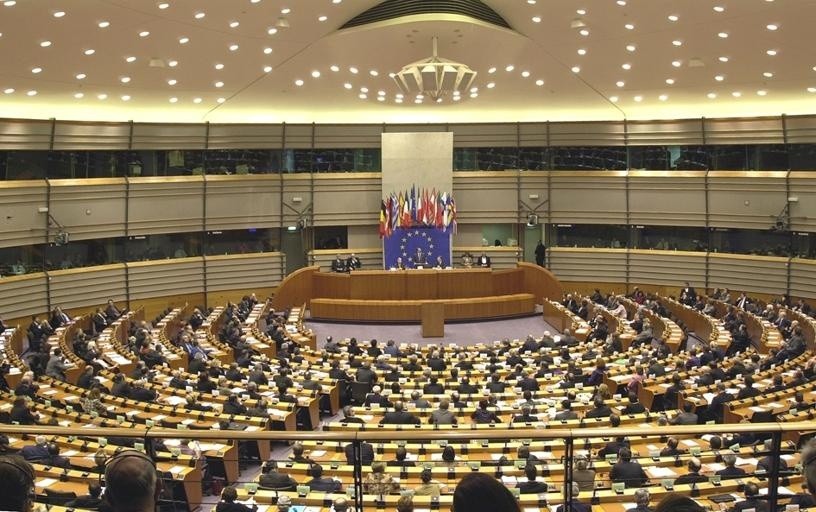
[0,293,816,512]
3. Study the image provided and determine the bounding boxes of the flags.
[379,183,457,241]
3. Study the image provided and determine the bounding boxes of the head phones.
[0,460,36,501]
[99,450,163,512]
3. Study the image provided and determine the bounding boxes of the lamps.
[392,34,478,102]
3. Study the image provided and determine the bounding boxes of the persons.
[478,251,490,267]
[347,253,360,271]
[535,239,546,267]
[0,237,269,276]
[414,248,425,262]
[461,252,474,266]
[330,328,575,512]
[562,281,816,512]
[1,293,355,512]
[333,254,347,271]
[558,235,816,259]
[395,257,406,270]
[436,255,446,269]
